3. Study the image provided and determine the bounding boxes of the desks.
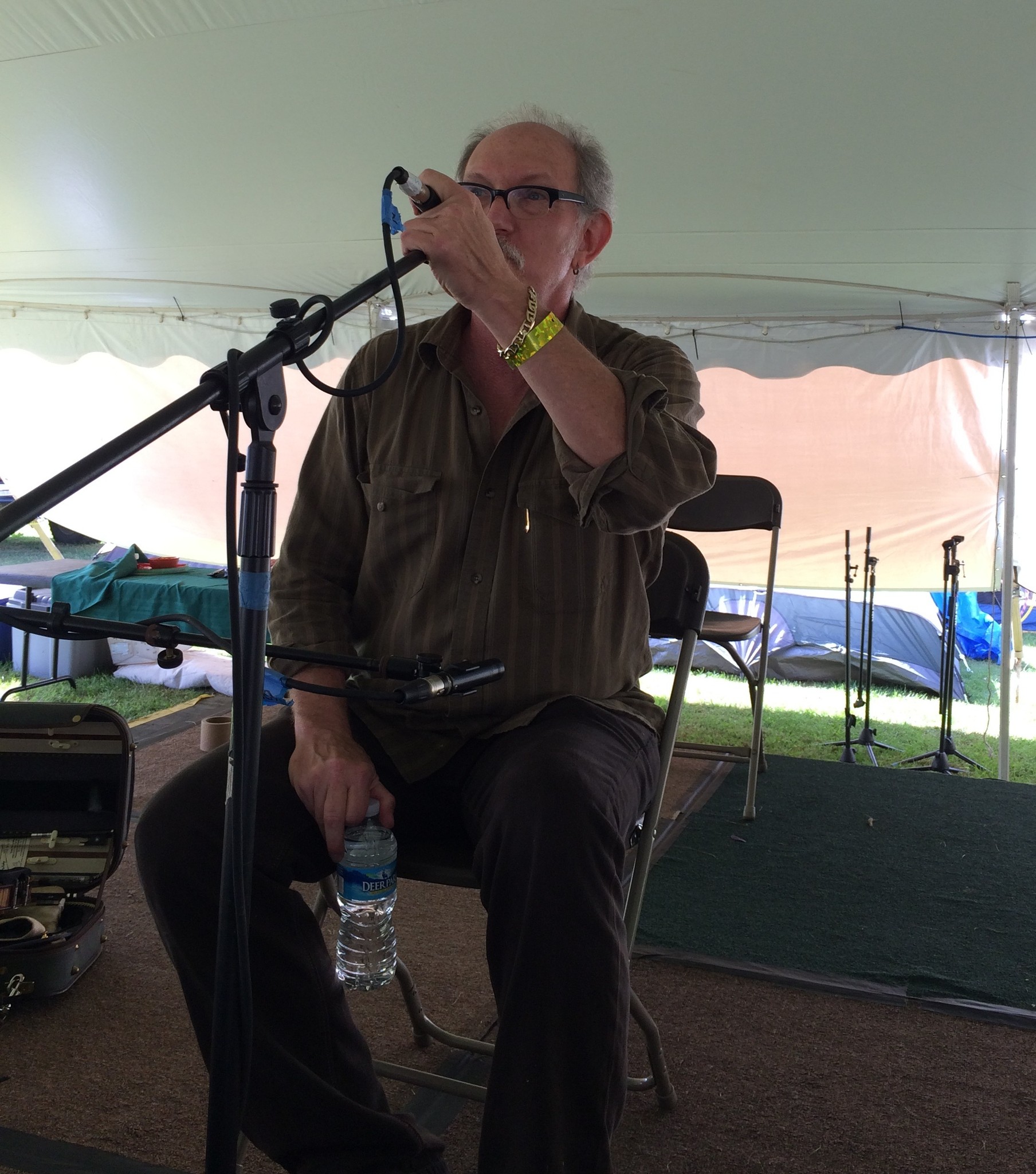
[0,559,272,705]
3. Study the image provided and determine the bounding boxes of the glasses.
[459,182,590,220]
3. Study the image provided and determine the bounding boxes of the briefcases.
[0,700,137,1006]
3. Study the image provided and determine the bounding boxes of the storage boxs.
[6,588,112,680]
[0,584,27,663]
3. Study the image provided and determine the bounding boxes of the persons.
[134,115,717,1174]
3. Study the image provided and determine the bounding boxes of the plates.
[137,563,191,570]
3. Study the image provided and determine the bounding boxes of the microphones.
[392,657,505,708]
[393,166,443,214]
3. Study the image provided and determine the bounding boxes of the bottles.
[333,799,400,989]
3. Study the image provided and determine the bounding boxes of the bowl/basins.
[148,557,180,569]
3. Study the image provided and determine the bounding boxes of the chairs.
[667,475,782,819]
[311,531,707,1110]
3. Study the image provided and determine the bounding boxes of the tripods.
[821,526,992,776]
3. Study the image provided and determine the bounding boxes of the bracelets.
[495,284,537,363]
[506,311,566,371]
